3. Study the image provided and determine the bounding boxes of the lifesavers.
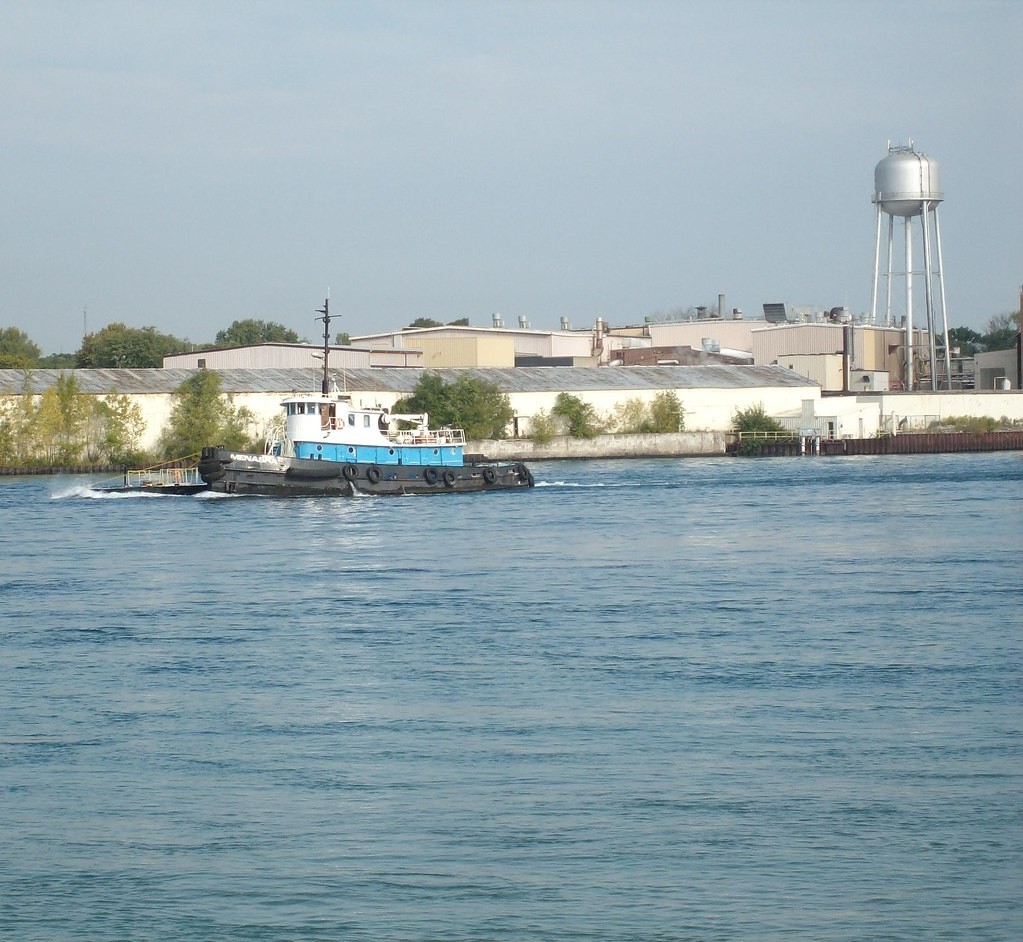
[425,467,440,484]
[367,467,382,484]
[198,461,227,483]
[444,470,457,486]
[483,468,497,483]
[343,464,359,480]
[334,417,345,430]
[518,464,530,481]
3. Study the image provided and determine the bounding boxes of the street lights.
[310,286,341,397]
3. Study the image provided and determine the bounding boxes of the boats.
[198,394,536,496]
[84,466,209,495]
